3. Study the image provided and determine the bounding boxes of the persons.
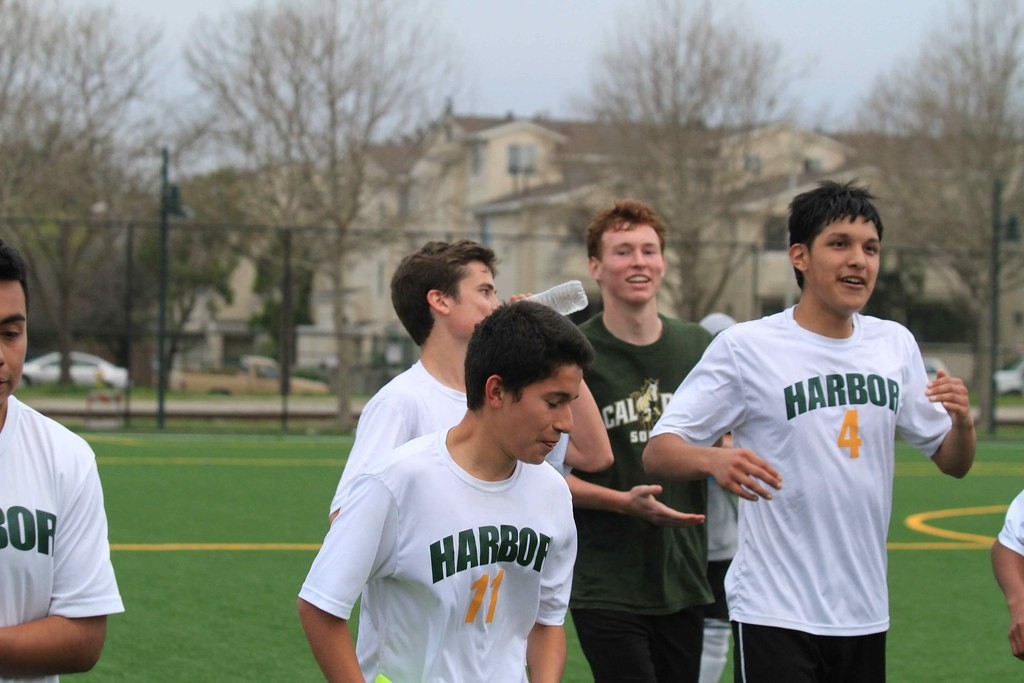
[295,202,741,683]
[991,488,1024,659]
[0,239,125,683]
[645,176,978,683]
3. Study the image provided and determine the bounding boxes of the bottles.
[516,280,588,316]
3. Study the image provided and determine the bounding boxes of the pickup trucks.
[171,351,334,399]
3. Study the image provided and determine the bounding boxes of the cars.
[14,350,130,390]
[921,356,951,386]
[991,352,1024,398]
